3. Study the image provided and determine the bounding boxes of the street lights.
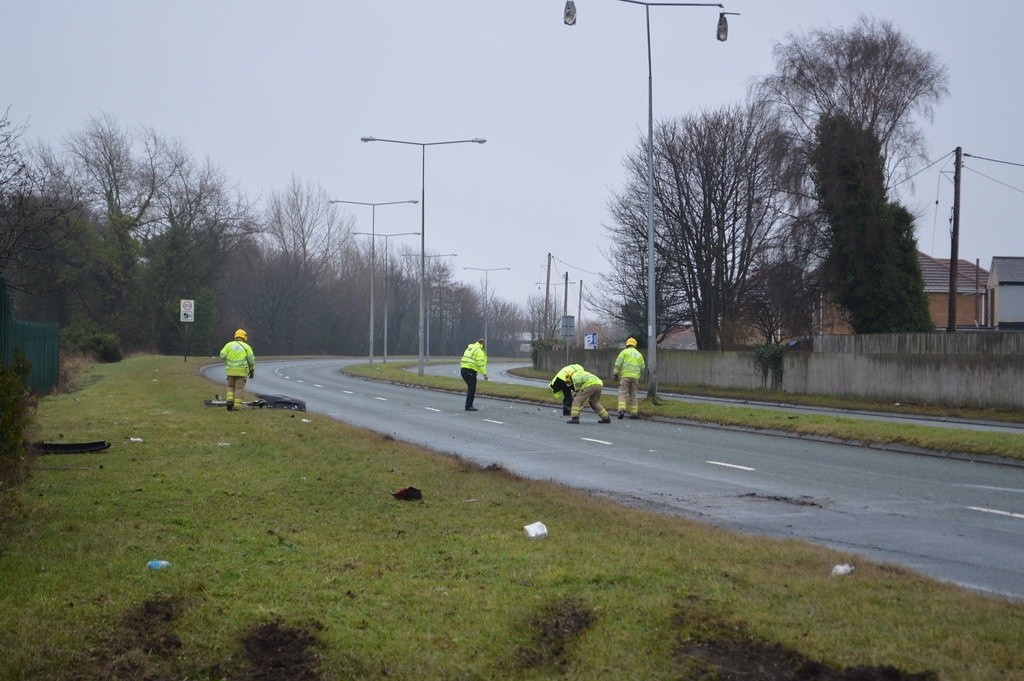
[562,0,743,401]
[402,254,459,363]
[352,232,422,363]
[534,281,578,340]
[328,198,420,369]
[605,279,628,309]
[463,267,511,353]
[359,136,487,377]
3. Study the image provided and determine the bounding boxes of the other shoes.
[629,415,638,419]
[234,409,240,411]
[598,419,610,423]
[566,418,580,424]
[465,408,477,411]
[618,412,624,419]
[227,402,232,411]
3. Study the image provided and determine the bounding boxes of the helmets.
[234,329,247,341]
[565,372,573,382]
[626,338,637,346]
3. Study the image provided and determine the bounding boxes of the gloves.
[248,370,254,379]
[484,376,488,381]
[614,376,618,381]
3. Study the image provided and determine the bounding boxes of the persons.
[613,337,646,420]
[549,364,611,424]
[219,329,256,411]
[460,338,488,411]
